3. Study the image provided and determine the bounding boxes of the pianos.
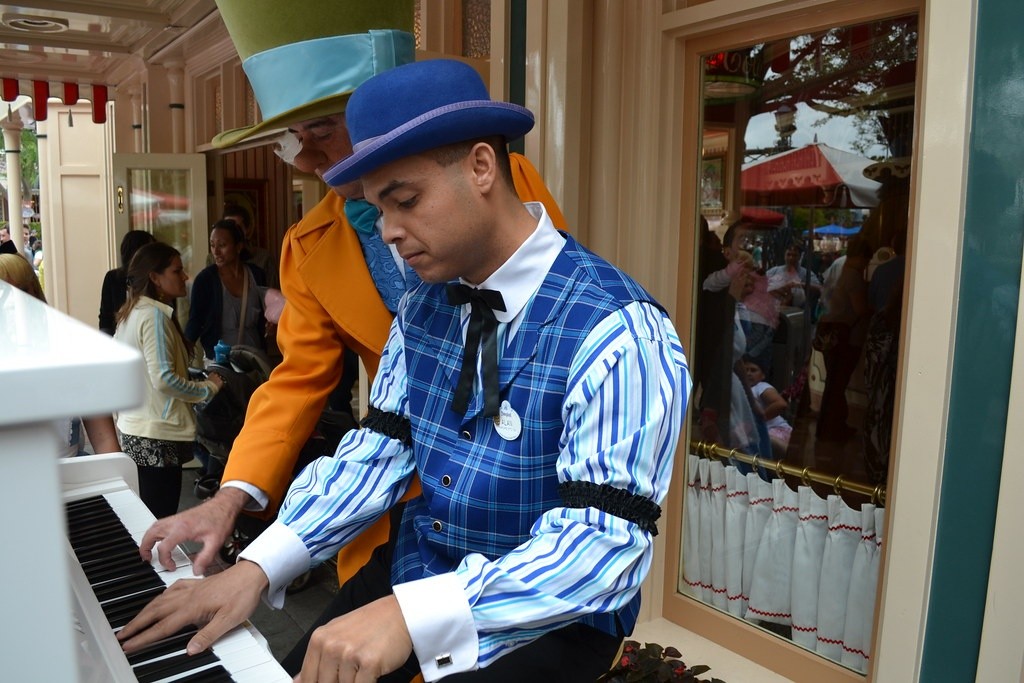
[0,278,295,683]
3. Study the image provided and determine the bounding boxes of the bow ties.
[446,281,507,419]
[343,197,384,233]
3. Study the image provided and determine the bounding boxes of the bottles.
[215,340,231,364]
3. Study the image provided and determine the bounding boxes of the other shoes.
[287,568,324,593]
[795,405,812,418]
[817,424,860,440]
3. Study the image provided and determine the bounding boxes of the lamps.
[774,100,798,140]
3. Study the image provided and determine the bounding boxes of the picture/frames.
[700,148,727,216]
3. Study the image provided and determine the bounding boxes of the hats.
[322,58,534,186]
[211,0,416,149]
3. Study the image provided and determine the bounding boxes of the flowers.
[616,640,727,683]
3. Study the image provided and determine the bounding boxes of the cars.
[131,186,192,237]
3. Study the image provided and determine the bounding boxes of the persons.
[139,0,569,586]
[97,230,178,336]
[0,224,121,455]
[116,59,695,683]
[110,242,223,518]
[180,209,277,495]
[699,123,912,484]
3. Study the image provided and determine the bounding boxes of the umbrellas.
[738,136,888,291]
